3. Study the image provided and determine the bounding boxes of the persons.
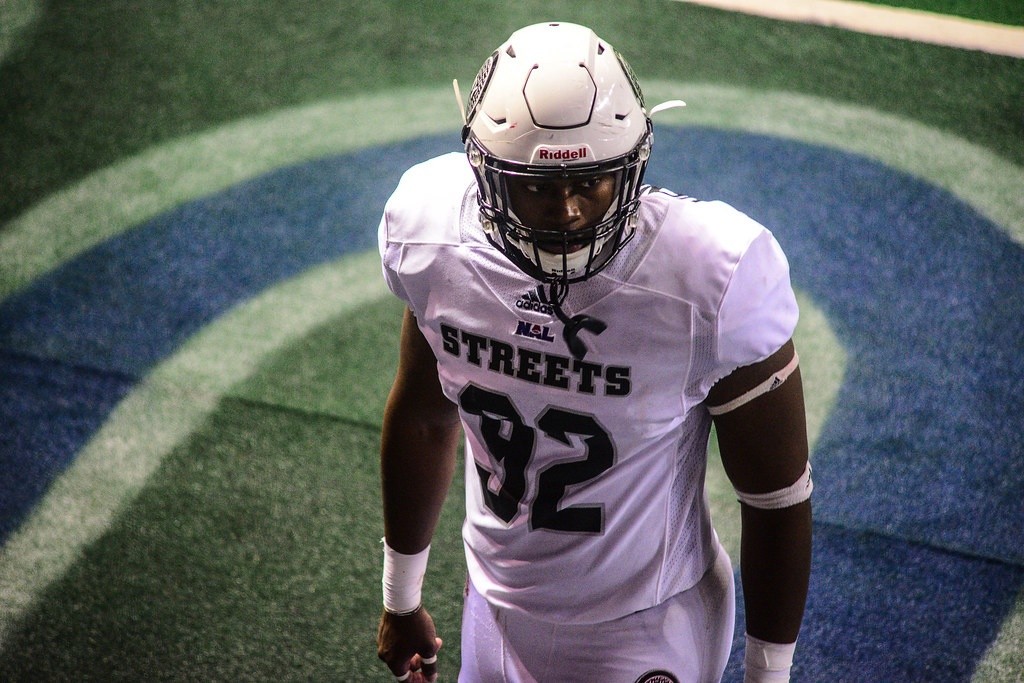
[378,22,813,683]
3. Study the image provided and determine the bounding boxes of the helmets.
[461,21,654,287]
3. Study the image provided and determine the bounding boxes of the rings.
[396,670,410,681]
[421,653,437,664]
